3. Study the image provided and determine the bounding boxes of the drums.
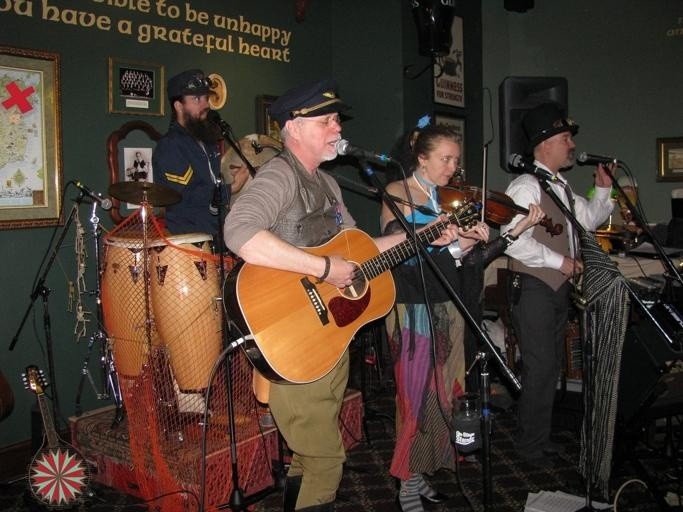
[148,232,224,394]
[98,228,170,379]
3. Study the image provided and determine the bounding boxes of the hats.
[268,78,352,127]
[166,69,215,99]
[522,102,579,153]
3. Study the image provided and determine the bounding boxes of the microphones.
[578,152,621,167]
[72,179,115,212]
[207,109,234,134]
[505,153,554,181]
[208,171,222,216]
[337,139,390,165]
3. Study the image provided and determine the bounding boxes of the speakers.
[501,72,567,171]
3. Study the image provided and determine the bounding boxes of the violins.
[436,180,563,237]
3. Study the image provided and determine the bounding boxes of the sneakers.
[523,449,553,469]
[542,440,562,454]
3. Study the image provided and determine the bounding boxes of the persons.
[127,151,148,181]
[380,114,547,512]
[120,69,152,95]
[154,69,249,231]
[497,101,618,466]
[221,81,463,512]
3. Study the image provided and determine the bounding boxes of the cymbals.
[108,181,181,207]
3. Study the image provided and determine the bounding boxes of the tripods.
[74,218,125,417]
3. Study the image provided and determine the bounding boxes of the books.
[523,487,612,511]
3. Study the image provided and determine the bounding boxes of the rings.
[536,215,541,220]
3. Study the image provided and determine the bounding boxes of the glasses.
[301,115,341,127]
[185,77,212,90]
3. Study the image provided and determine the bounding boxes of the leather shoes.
[419,491,448,505]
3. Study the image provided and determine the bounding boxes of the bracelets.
[315,253,331,285]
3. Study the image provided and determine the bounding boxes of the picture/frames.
[107,58,168,118]
[0,45,67,230]
[107,121,169,226]
[431,113,467,184]
[655,135,682,183]
[429,14,468,112]
[252,90,277,141]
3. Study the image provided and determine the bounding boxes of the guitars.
[223,197,483,385]
